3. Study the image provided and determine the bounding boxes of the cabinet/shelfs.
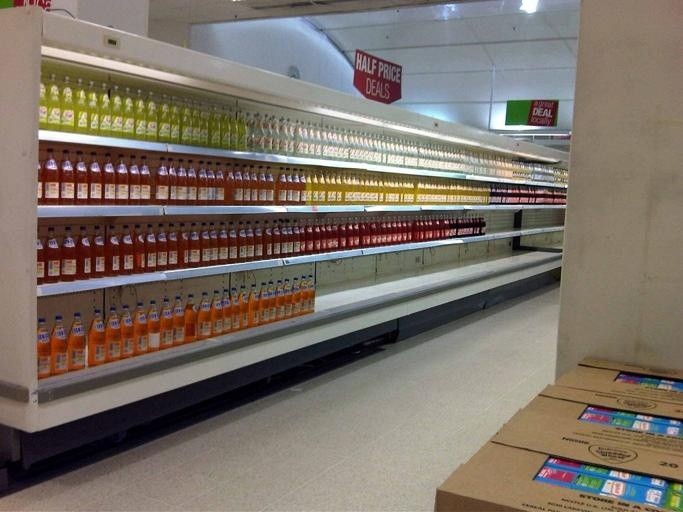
[1,7,573,472]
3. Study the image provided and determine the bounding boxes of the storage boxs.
[434,356,682,511]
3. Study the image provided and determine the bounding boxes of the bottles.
[38,72,569,210]
[34,274,315,382]
[38,212,487,286]
